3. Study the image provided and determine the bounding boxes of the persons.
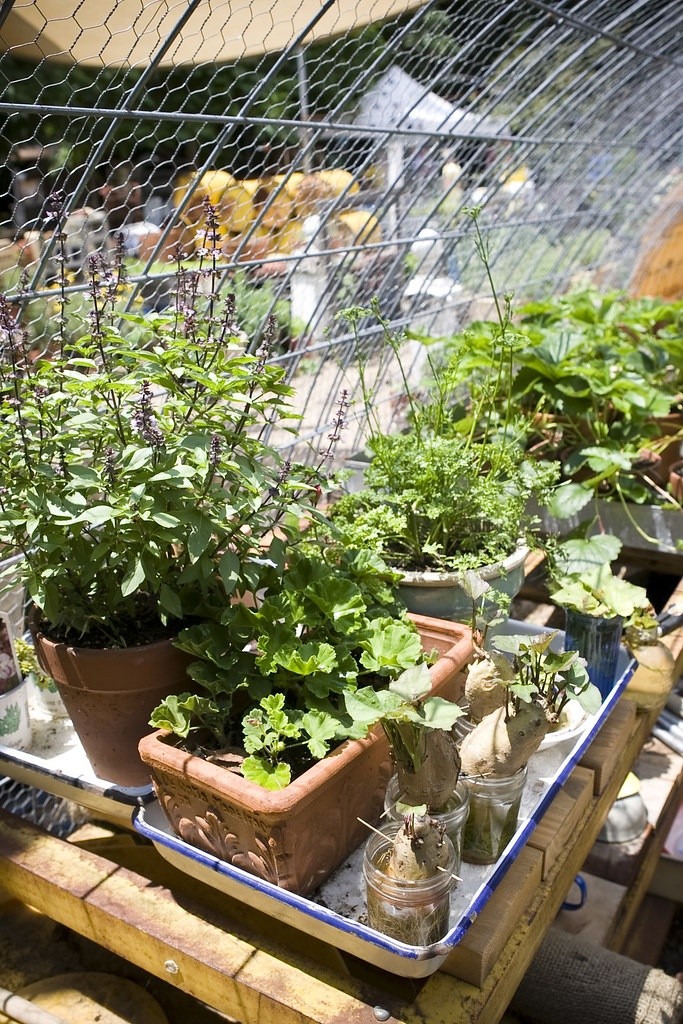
[486,151,539,218]
[102,147,143,230]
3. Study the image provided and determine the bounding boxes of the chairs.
[45,211,109,275]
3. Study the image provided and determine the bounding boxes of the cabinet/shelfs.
[0,544,682,1024]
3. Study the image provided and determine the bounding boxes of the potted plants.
[0,193,683,945]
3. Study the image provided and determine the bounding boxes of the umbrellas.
[311,65,509,238]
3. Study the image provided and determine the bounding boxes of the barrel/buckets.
[10,140,380,261]
